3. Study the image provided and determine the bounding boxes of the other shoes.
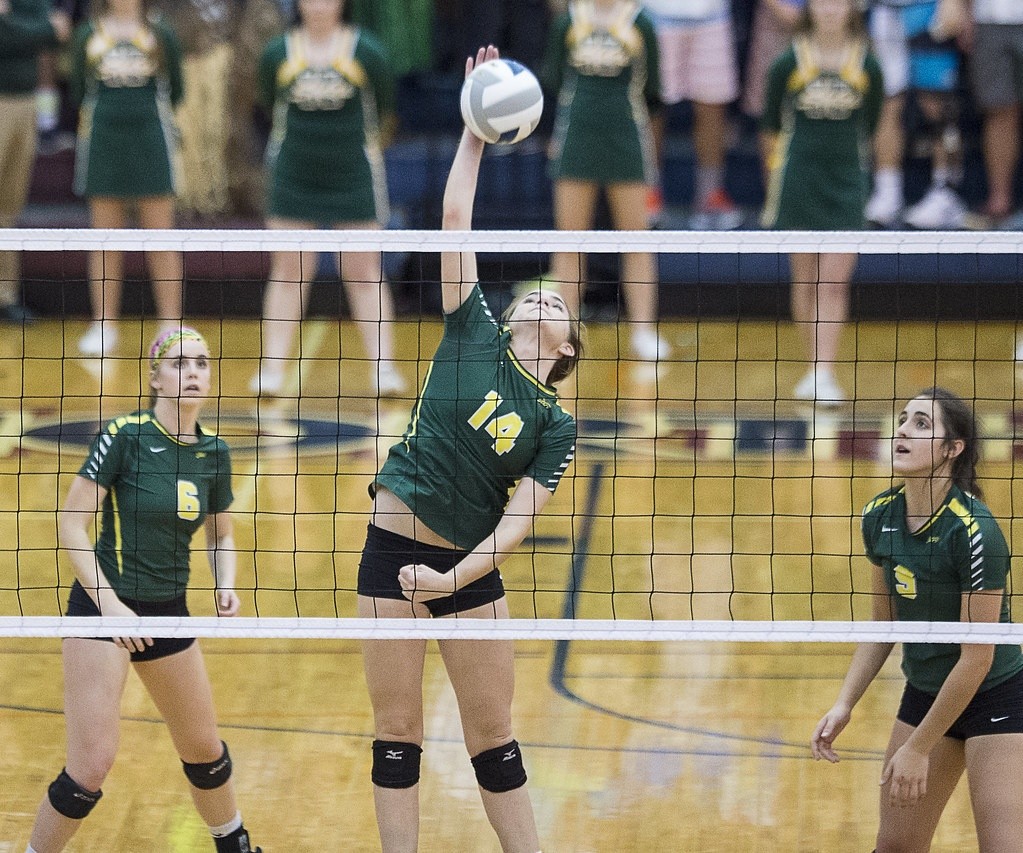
[77,325,120,354]
[961,200,1015,233]
[899,187,965,229]
[372,368,407,398]
[688,192,750,230]
[634,332,670,360]
[249,367,288,396]
[793,370,844,409]
[862,184,905,229]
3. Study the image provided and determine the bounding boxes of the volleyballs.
[459,59,545,145]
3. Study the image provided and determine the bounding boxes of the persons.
[30,324,273,852]
[355,43,588,851]
[810,387,1023,853]
[0,0,1023,411]
